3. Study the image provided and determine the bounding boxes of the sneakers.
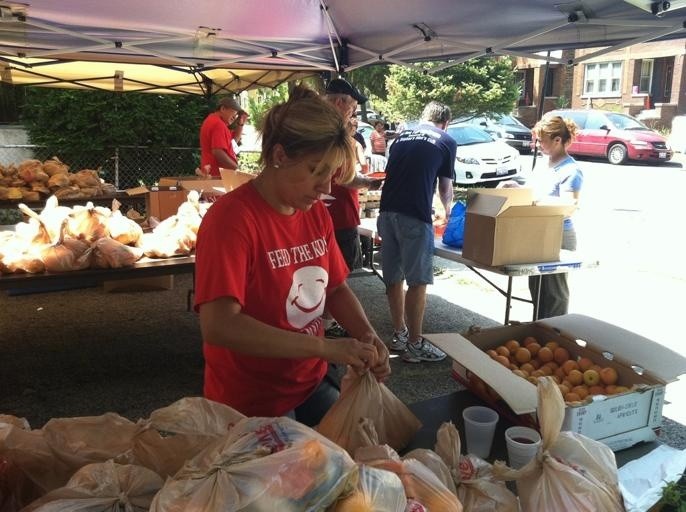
[399,339,447,362]
[324,322,347,338]
[388,328,410,350]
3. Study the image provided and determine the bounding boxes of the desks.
[0,191,145,210]
[356,218,581,326]
[313,388,686,511]
[0,253,196,292]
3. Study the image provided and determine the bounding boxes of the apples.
[300,440,327,470]
[337,492,374,512]
[485,335,630,406]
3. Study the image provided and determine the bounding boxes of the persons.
[351,120,386,174]
[319,78,386,338]
[523,115,583,321]
[377,101,456,364]
[199,97,248,178]
[192,96,392,425]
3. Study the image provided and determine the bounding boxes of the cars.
[344,105,396,161]
[438,121,521,190]
[470,108,535,152]
[533,104,673,167]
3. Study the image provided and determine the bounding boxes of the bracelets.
[237,122,244,126]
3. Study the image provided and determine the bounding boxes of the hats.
[327,79,366,104]
[220,98,242,110]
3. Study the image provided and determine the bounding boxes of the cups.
[462,405,499,459]
[505,425,541,468]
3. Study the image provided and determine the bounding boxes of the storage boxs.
[125,168,257,221]
[463,187,577,267]
[104,274,174,293]
[417,313,685,453]
